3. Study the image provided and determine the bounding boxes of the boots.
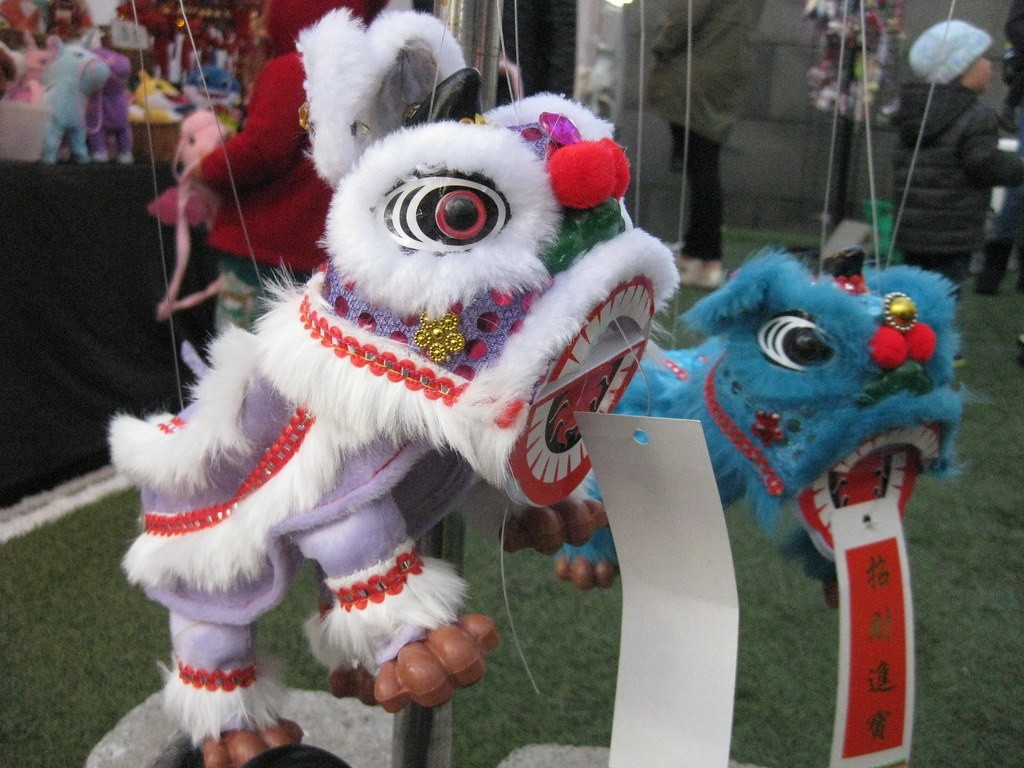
[997,99,1017,133]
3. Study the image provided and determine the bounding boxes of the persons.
[193,0,363,334]
[650,1,766,289]
[893,0,1023,293]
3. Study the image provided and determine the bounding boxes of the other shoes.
[673,257,724,290]
[972,280,993,295]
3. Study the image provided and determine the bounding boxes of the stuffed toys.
[178,49,242,119]
[10,27,134,164]
[129,69,184,125]
[151,110,236,228]
[550,247,962,590]
[106,9,676,767]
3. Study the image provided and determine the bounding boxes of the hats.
[909,20,992,86]
